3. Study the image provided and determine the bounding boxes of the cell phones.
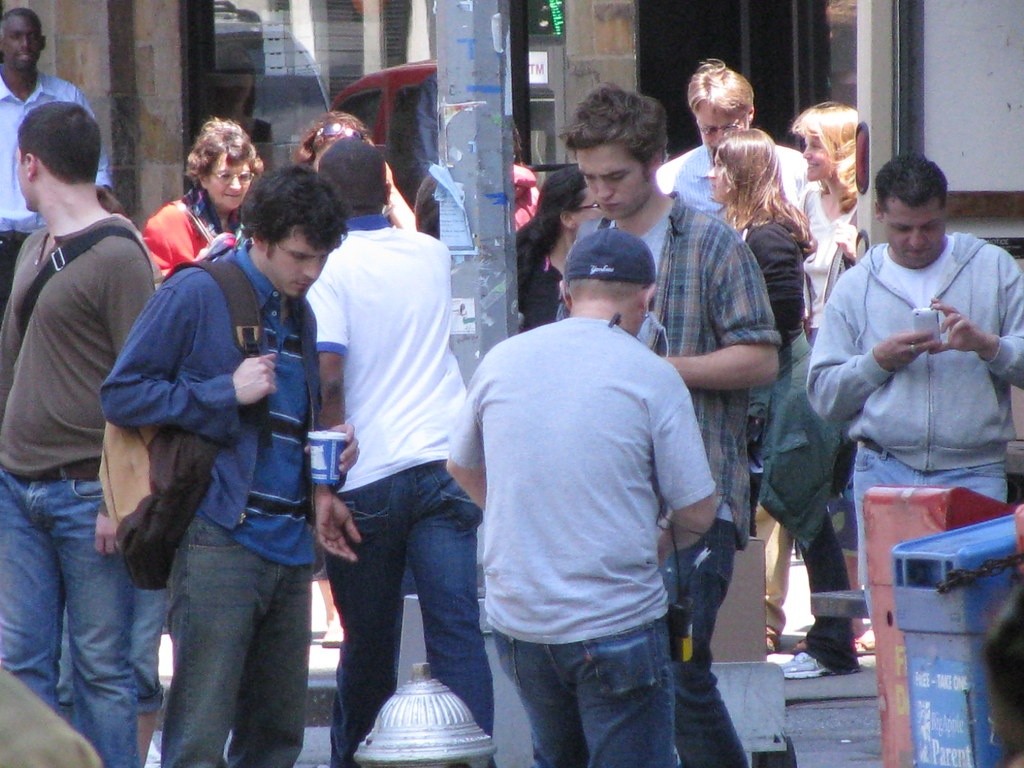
[912,307,941,341]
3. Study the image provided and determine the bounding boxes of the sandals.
[790,637,811,653]
[764,624,780,654]
[854,624,877,654]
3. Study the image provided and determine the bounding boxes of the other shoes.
[309,619,344,648]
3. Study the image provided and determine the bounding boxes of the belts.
[15,459,97,480]
[0,231,30,242]
[861,437,895,459]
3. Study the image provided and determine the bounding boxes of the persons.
[789,106,876,653]
[1,8,130,327]
[521,168,604,330]
[449,227,716,768]
[709,129,859,679]
[0,101,155,767]
[56,189,166,767]
[144,118,264,284]
[313,110,415,649]
[564,82,778,768]
[102,165,347,768]
[807,154,1023,614]
[656,61,807,225]
[304,136,496,767]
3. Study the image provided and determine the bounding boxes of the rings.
[911,345,918,356]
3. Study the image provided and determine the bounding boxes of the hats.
[563,227,656,312]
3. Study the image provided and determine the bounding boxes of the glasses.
[561,200,601,213]
[318,121,361,140]
[206,170,254,185]
[698,111,747,136]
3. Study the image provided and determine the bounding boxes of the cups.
[308,431,347,486]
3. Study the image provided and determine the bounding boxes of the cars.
[338,58,559,226]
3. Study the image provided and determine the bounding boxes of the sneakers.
[778,652,859,680]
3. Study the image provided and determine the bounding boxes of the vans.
[213,17,333,174]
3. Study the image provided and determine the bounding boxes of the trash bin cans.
[860,483,1024,768]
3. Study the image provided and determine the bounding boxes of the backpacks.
[114,256,263,592]
